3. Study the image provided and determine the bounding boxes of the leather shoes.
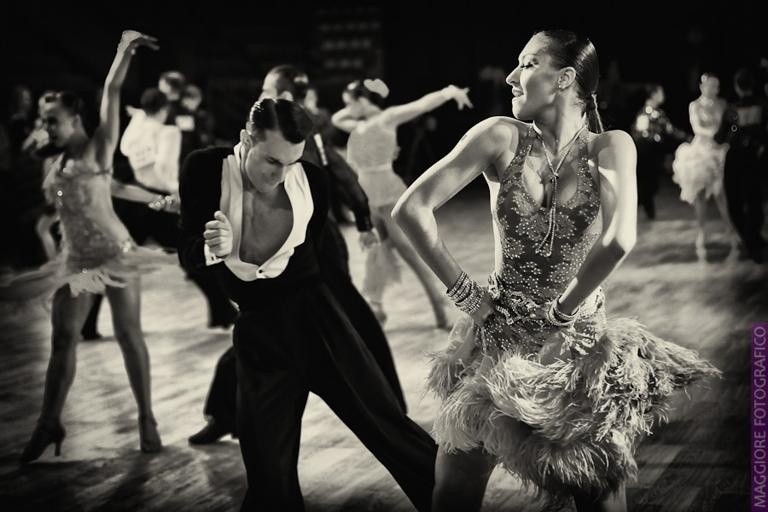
[189,421,238,443]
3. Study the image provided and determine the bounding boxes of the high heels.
[19,420,65,463]
[140,417,160,452]
